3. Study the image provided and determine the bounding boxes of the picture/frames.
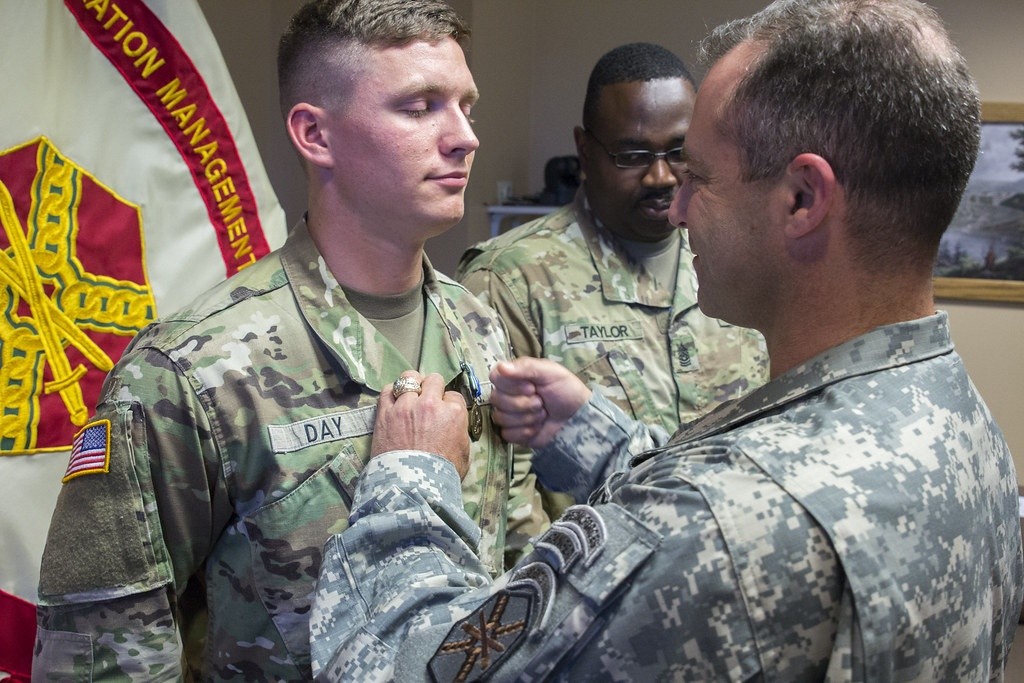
[931,102,1024,303]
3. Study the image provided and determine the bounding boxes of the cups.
[497,181,513,206]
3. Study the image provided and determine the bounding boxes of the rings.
[391,375,422,399]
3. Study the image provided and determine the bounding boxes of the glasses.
[584,122,685,169]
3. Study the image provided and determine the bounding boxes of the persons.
[450,41,770,526]
[310,1,1024,683]
[30,1,556,683]
